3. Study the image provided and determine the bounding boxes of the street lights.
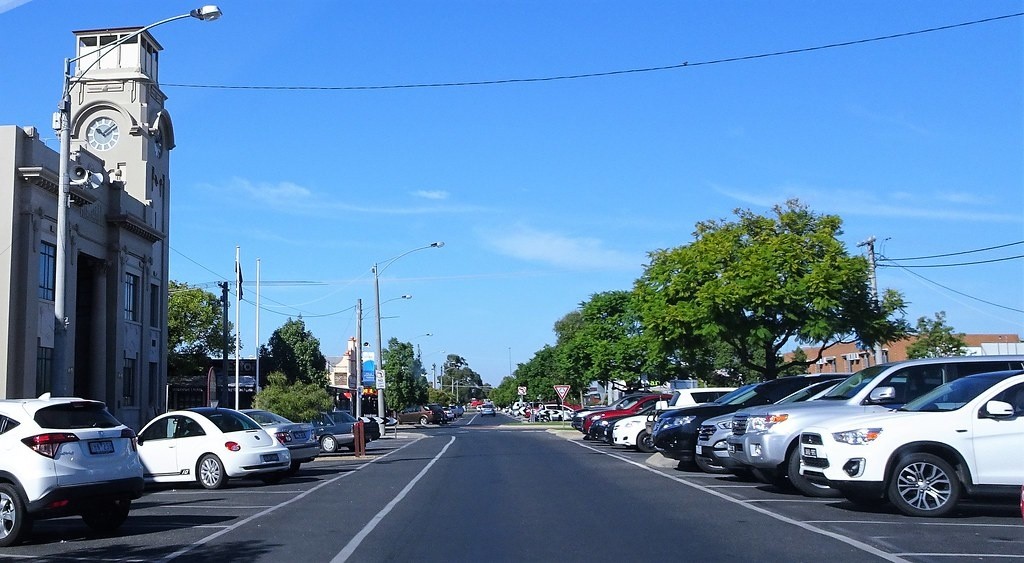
[373,241,447,437]
[49,5,224,397]
[356,294,412,418]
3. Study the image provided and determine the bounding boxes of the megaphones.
[69,165,87,185]
[86,170,104,189]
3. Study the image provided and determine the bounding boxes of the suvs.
[0,392,146,548]
[724,354,1024,500]
[650,373,853,467]
[396,402,446,426]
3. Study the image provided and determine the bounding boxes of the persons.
[526,406,531,417]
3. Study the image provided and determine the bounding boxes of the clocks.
[86,117,119,151]
[153,126,162,159]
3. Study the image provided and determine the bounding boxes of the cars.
[588,399,670,445]
[503,400,577,422]
[476,404,496,417]
[667,385,738,409]
[797,370,1024,517]
[611,404,656,454]
[308,411,382,453]
[443,405,464,421]
[571,393,653,431]
[582,394,675,437]
[131,406,292,491]
[695,376,906,487]
[231,409,321,473]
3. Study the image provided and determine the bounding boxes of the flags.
[235,261,243,300]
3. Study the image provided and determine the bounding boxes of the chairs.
[185,422,203,436]
